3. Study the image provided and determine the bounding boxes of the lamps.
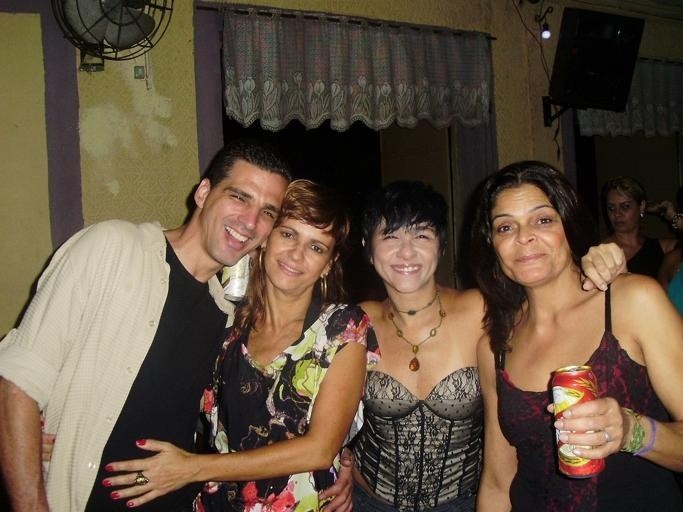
[541,19,551,40]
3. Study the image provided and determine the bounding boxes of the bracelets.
[620,407,645,453]
[672,214,683,229]
[634,416,656,455]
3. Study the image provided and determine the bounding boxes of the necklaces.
[384,289,445,371]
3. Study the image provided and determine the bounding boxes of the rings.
[604,429,611,444]
[134,471,149,486]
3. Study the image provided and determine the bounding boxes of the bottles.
[551,366,604,479]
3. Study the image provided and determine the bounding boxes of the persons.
[605,177,683,291]
[340,179,487,512]
[459,160,683,512]
[40,178,382,512]
[1,141,290,511]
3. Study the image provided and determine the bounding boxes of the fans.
[49,1,174,62]
